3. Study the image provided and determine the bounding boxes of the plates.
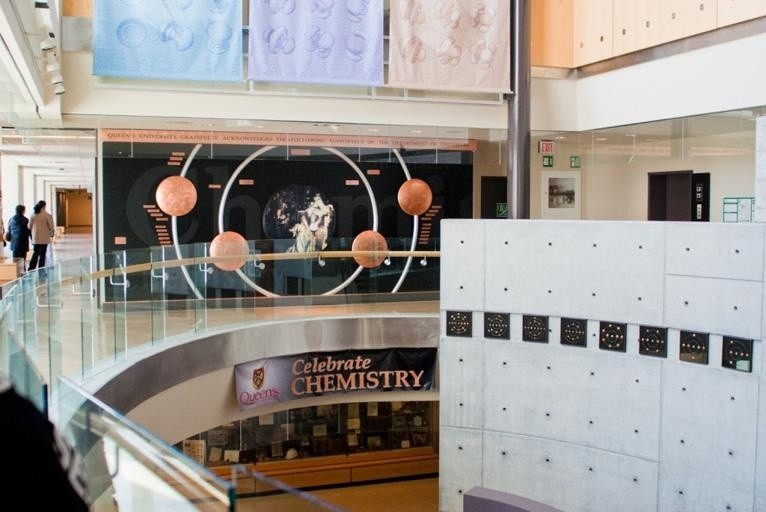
[231,399,433,468]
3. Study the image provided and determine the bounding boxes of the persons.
[4,205,31,271]
[28,201,55,280]
[0,378,89,512]
